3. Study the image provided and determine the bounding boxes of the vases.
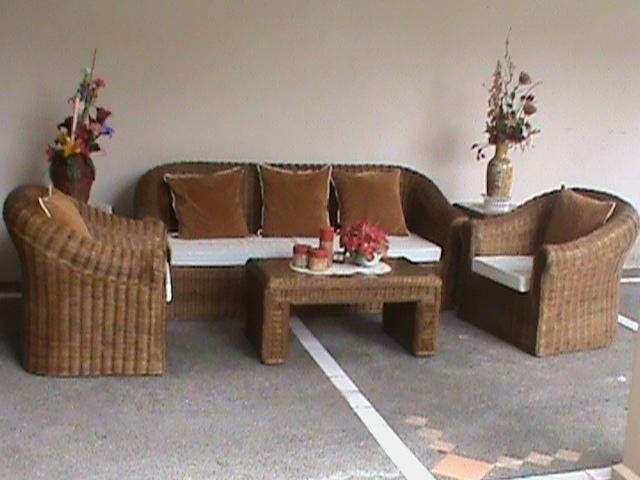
[48,151,97,204]
[481,141,514,209]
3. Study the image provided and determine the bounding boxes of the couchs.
[134,160,470,322]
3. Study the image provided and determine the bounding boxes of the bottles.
[320,226,334,267]
[308,247,329,272]
[292,244,312,268]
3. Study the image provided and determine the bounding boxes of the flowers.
[470,24,545,163]
[44,47,116,182]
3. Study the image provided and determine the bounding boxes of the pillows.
[162,162,410,243]
[545,184,616,244]
[36,185,96,243]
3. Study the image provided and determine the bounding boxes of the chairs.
[460,187,640,358]
[3,183,172,378]
